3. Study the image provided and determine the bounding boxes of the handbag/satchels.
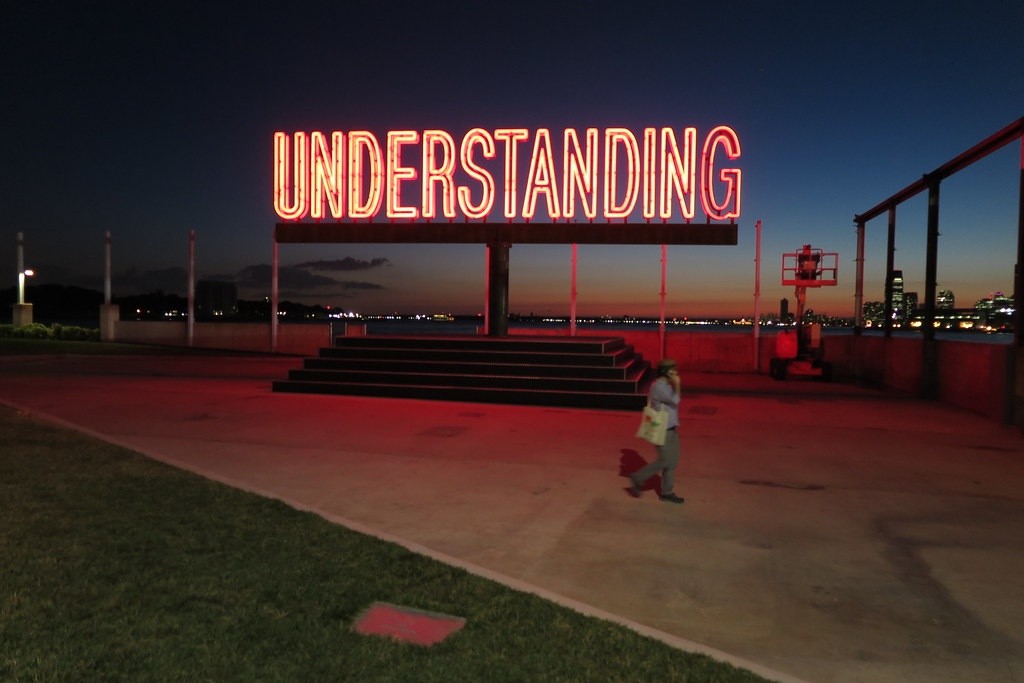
[636,382,668,446]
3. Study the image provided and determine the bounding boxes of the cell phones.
[666,373,673,378]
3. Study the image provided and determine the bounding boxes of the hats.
[658,359,676,370]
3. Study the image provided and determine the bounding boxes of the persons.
[628,360,685,503]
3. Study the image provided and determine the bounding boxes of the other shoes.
[626,476,640,498]
[660,493,684,504]
